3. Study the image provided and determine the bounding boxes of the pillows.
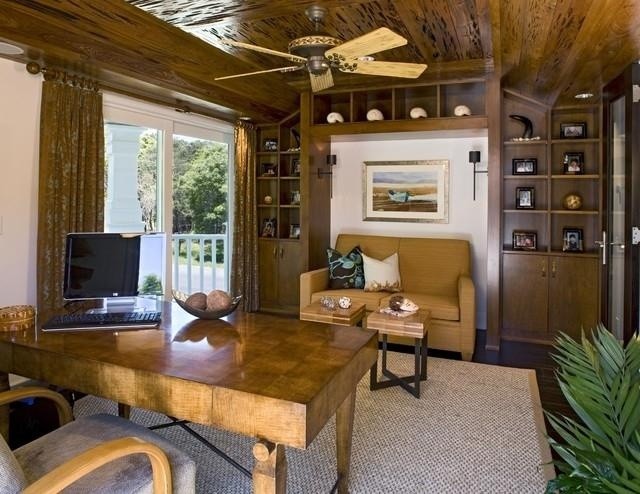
[325,245,365,290]
[356,251,403,292]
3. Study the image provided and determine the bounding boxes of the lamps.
[317,154,337,198]
[468,150,488,200]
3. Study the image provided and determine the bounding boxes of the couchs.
[299,234,476,361]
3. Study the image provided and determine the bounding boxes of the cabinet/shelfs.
[304,71,491,132]
[501,87,602,258]
[500,251,601,349]
[255,239,302,316]
[255,111,301,238]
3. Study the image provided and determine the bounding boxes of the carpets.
[73,347,561,494]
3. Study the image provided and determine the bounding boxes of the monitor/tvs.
[63,233,167,314]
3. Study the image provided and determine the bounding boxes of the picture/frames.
[361,159,450,224]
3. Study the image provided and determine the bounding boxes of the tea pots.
[319,295,334,308]
[338,296,352,310]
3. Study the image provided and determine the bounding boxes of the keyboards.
[41,312,162,332]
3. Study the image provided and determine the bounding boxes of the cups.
[327,300,335,311]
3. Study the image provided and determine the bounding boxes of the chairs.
[0,386,198,493]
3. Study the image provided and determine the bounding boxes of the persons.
[568,157,581,172]
[262,223,272,237]
[566,233,578,248]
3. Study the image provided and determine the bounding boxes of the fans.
[213,0,428,93]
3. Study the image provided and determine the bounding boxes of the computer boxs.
[8,380,74,451]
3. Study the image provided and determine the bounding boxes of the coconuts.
[186,289,232,313]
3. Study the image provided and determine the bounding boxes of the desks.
[0,302,378,493]
